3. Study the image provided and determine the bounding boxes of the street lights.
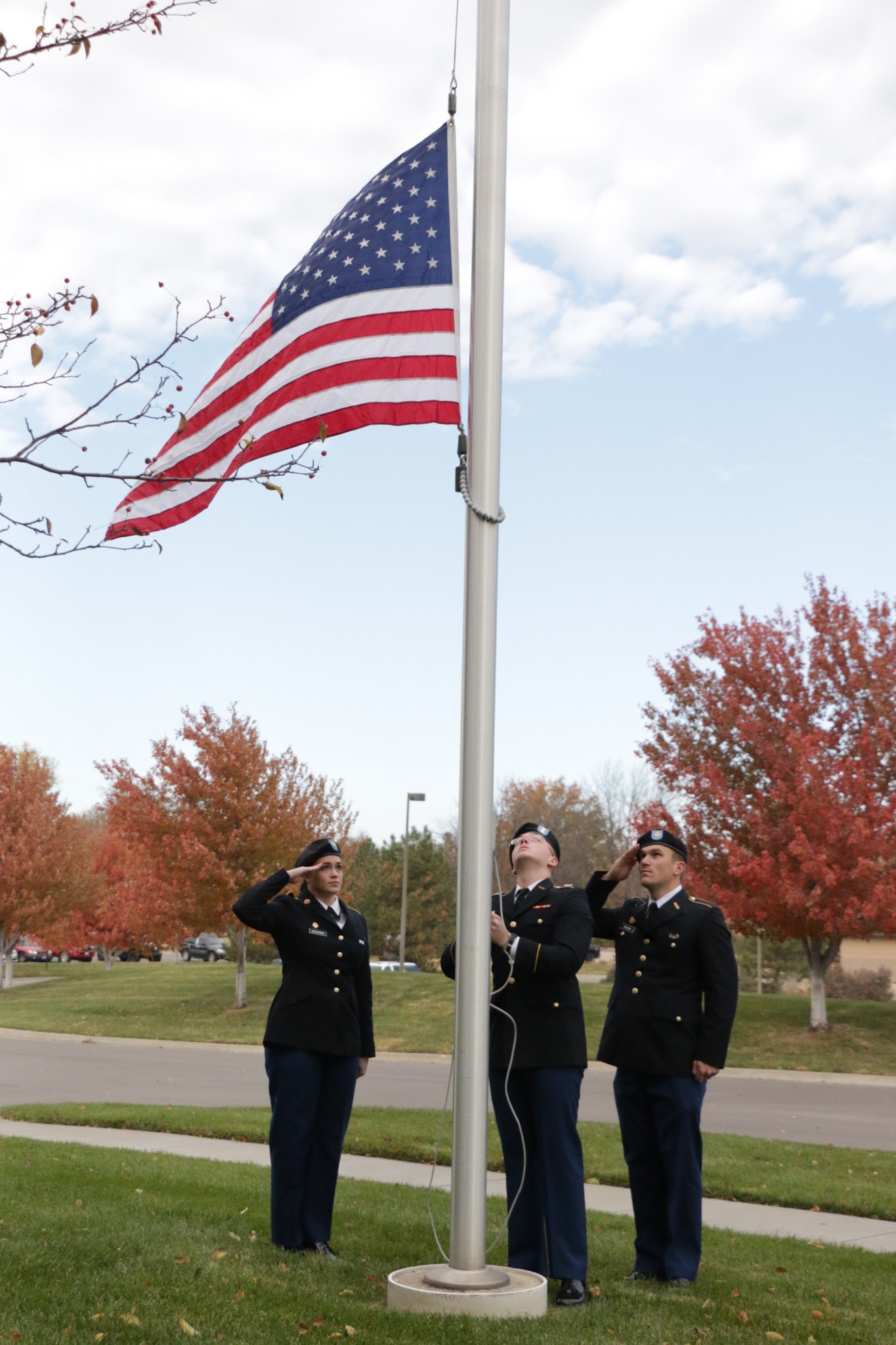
[399,793,425,972]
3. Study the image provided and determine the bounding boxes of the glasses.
[510,835,555,857]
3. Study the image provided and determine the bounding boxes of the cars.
[43,934,96,962]
[4,933,53,963]
[369,961,421,972]
[96,933,162,963]
[178,932,235,963]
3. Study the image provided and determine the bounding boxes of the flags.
[105,123,458,541]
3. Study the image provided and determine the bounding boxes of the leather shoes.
[556,1278,587,1305]
[283,1246,317,1258]
[622,1271,646,1281]
[664,1277,689,1288]
[310,1239,356,1271]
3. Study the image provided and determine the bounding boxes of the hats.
[510,822,560,869]
[295,838,341,868]
[637,829,687,863]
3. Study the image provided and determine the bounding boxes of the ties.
[327,906,337,923]
[513,888,529,912]
[644,903,657,920]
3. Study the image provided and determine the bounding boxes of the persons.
[585,830,738,1282]
[231,838,376,1253]
[441,823,593,1304]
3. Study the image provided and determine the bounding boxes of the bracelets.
[506,933,517,953]
